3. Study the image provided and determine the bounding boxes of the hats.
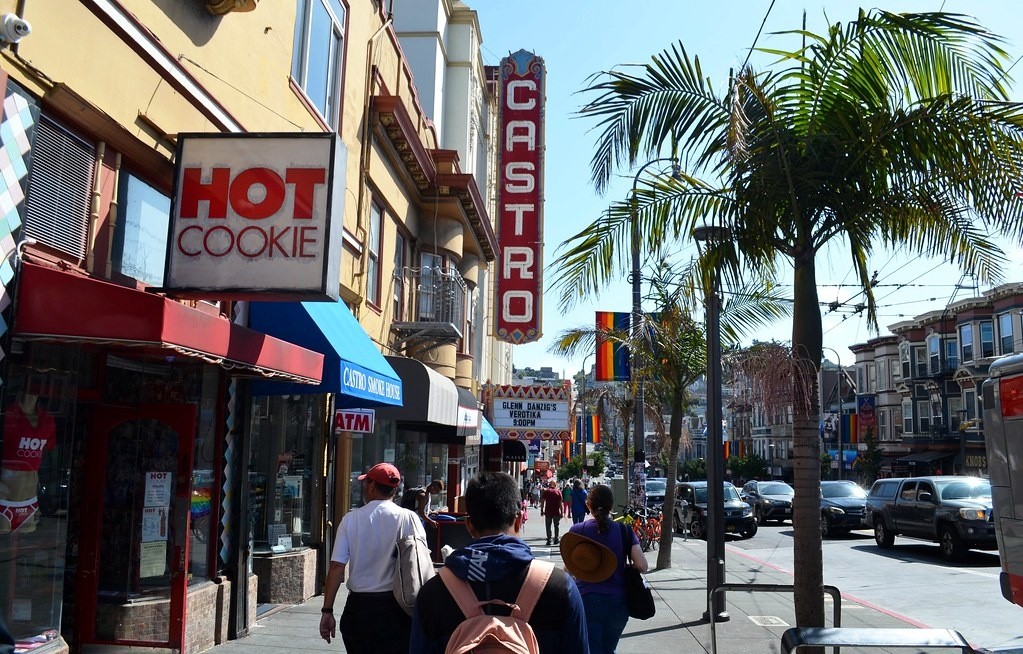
[560,532,617,583]
[536,479,539,482]
[358,463,401,487]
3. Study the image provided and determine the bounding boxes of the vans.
[865,475,999,560]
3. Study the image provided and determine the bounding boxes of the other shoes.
[546,540,551,545]
[554,537,560,545]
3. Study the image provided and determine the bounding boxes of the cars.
[790,480,869,537]
[37,479,69,515]
[646,480,668,506]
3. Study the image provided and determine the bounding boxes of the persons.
[564,485,648,654]
[0,387,56,533]
[569,479,588,525]
[541,481,564,546]
[527,477,591,518]
[414,480,443,529]
[320,463,435,654]
[408,473,590,654]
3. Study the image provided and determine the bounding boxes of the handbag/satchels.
[584,504,590,515]
[619,523,655,620]
[392,535,436,618]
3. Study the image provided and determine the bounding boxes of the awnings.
[15,259,325,388]
[455,386,479,438]
[250,293,404,409]
[481,415,499,445]
[374,355,459,430]
[896,449,959,464]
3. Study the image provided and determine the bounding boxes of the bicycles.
[633,512,646,549]
[610,504,634,526]
[654,507,674,544]
[644,509,656,550]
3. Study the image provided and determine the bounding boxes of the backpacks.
[401,485,429,512]
[438,560,555,654]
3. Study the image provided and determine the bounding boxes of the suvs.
[740,480,795,525]
[673,481,757,539]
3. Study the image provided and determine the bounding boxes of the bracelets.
[321,607,333,613]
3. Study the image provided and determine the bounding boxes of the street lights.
[691,225,732,620]
